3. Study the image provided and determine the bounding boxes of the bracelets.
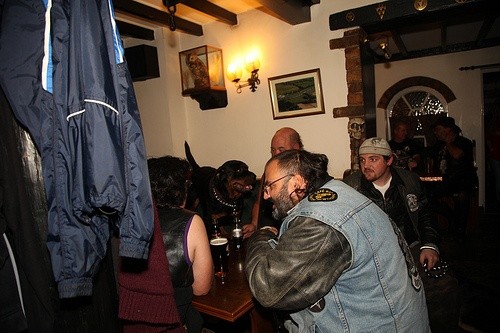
[423,259,448,278]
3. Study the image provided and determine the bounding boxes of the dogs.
[180,138,260,237]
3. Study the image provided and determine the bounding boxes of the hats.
[359,138,391,156]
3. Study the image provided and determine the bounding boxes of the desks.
[191,259,275,333]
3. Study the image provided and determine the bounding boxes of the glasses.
[185,180,192,187]
[262,174,294,192]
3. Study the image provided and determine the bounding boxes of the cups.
[209,238,229,279]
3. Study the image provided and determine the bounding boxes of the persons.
[239,148,432,333]
[386,121,428,183]
[339,136,458,333]
[241,128,305,237]
[148,156,214,333]
[427,116,481,241]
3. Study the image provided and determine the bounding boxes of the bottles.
[231,212,245,260]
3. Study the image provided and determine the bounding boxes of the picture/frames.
[267,68,326,121]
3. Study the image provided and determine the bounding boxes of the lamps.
[226,56,261,94]
[380,35,391,59]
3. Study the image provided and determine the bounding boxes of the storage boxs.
[178,45,225,97]
